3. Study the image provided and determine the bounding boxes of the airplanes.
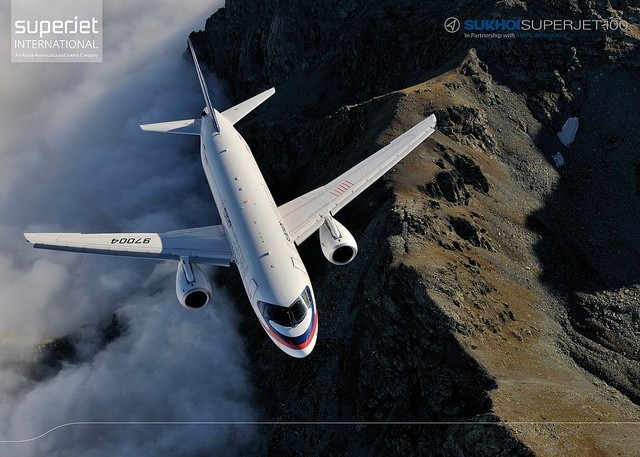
[24,37,439,358]
